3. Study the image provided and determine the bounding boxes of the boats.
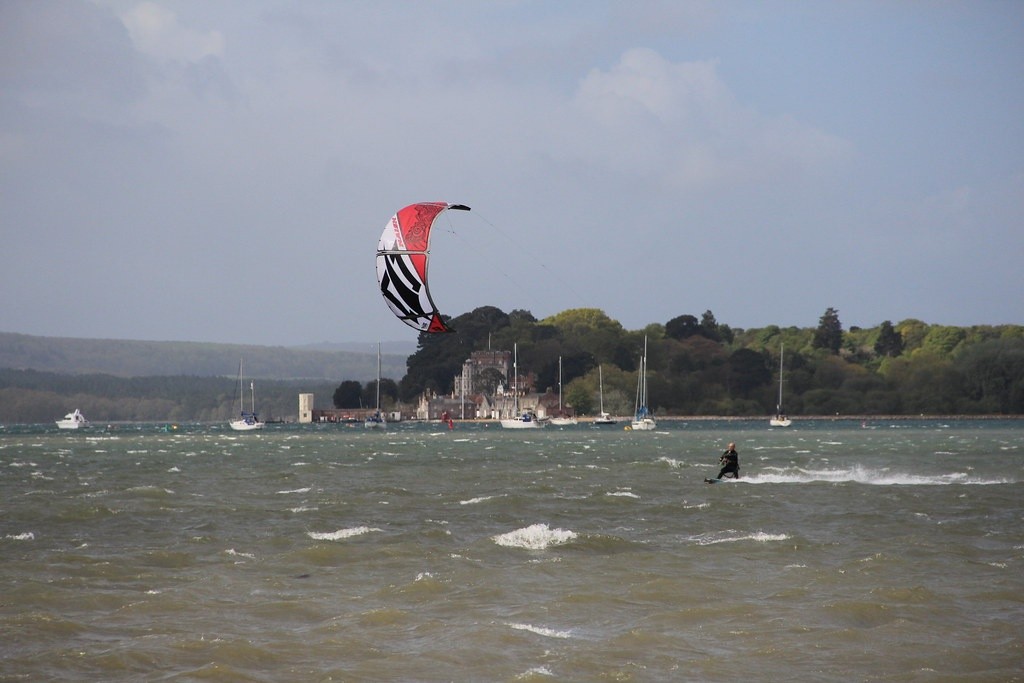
[55,408,94,430]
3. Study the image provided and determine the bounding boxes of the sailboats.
[594,365,619,425]
[548,356,578,426]
[362,341,388,431]
[769,342,792,427]
[226,356,264,431]
[624,335,658,432]
[498,343,547,430]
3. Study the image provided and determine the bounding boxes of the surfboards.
[704,478,748,484]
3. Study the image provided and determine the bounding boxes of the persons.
[717,443,739,479]
